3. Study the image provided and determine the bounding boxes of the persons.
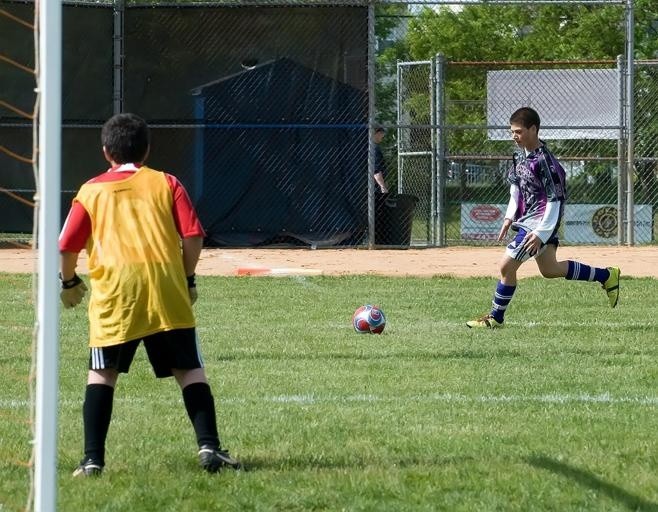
[466,107,621,329]
[57,113,240,479]
[374,127,388,193]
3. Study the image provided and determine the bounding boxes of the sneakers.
[466,313,505,330]
[72,455,104,477]
[601,265,621,308]
[197,443,242,473]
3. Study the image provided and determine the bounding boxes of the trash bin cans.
[374,191,419,249]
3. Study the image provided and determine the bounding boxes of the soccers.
[352,304,386,335]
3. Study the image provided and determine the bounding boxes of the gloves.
[186,272,199,306]
[57,270,89,308]
